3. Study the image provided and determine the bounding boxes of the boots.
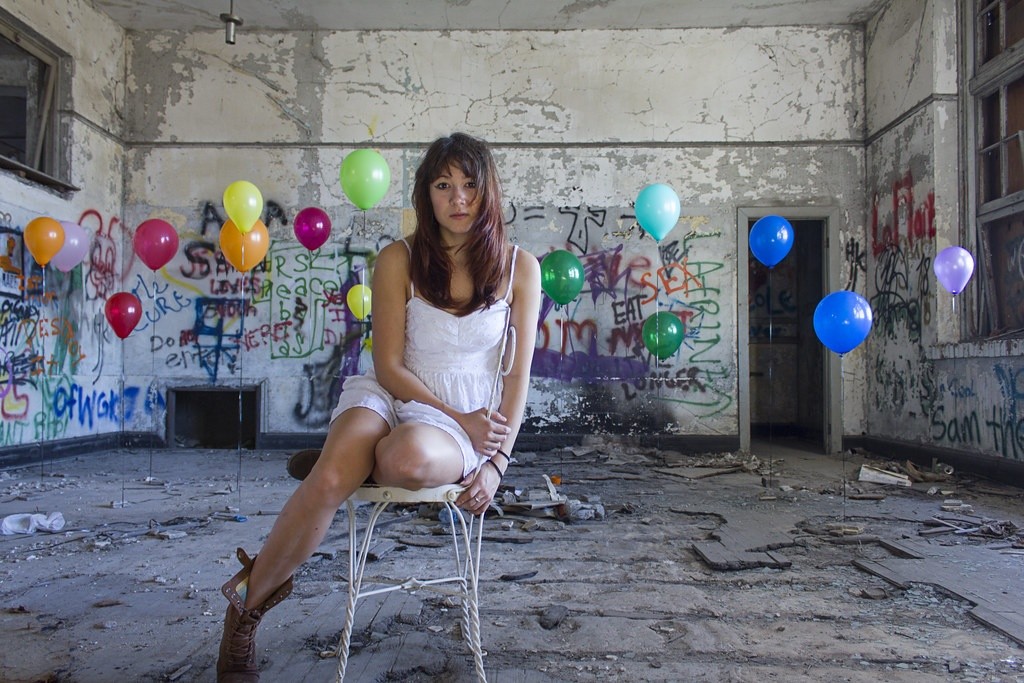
[215,546,294,682]
[287,449,380,488]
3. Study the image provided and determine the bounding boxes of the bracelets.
[488,446,510,480]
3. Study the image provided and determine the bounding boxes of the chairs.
[338,304,517,681]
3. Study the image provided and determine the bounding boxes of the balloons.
[749,214,795,270]
[23,216,66,268]
[539,249,586,307]
[132,217,180,273]
[634,182,681,244]
[932,246,976,297]
[346,283,372,320]
[50,219,90,273]
[104,291,143,341]
[292,206,333,252]
[813,290,873,357]
[218,217,271,276]
[641,310,684,363]
[222,178,265,236]
[339,148,391,213]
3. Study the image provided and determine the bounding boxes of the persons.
[216,132,543,683]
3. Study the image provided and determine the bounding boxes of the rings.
[474,497,480,502]
